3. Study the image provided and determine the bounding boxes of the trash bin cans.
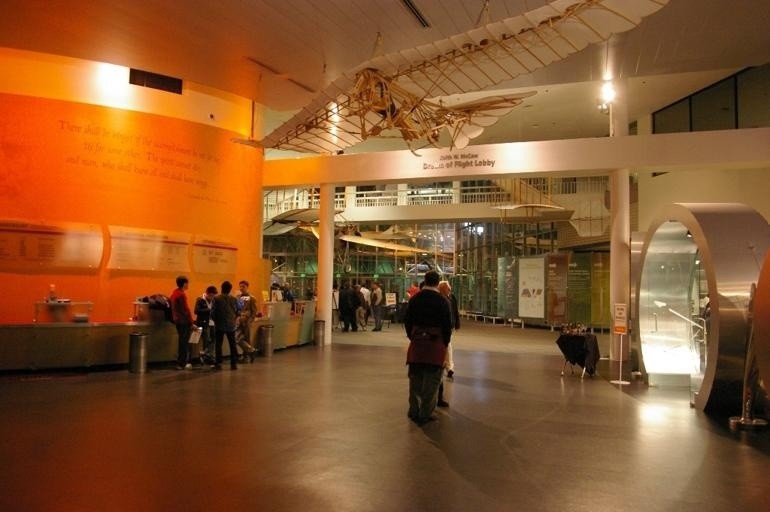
[314,320,325,349]
[128,332,148,374]
[259,325,274,358]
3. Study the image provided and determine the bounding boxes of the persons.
[270,283,293,301]
[168,275,199,370]
[193,280,258,373]
[330,281,383,331]
[403,271,461,425]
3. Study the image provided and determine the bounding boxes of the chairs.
[381,304,404,328]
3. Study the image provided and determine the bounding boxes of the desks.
[557,333,601,379]
